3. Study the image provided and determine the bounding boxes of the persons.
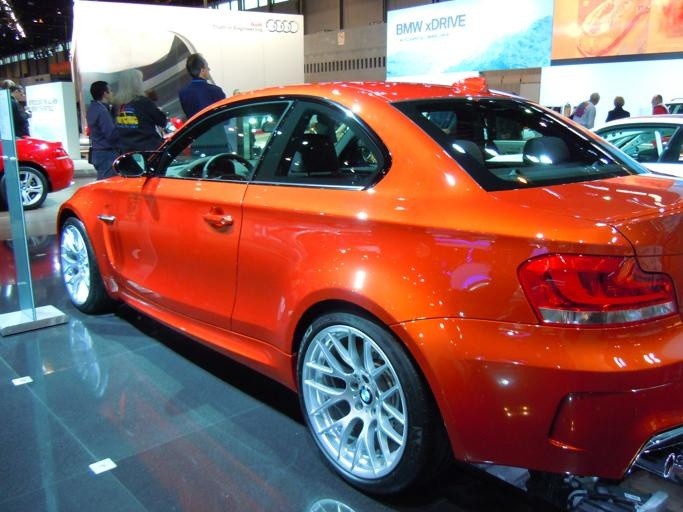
[652,95,669,114]
[2,79,32,137]
[606,97,630,122]
[110,70,168,155]
[573,92,599,129]
[179,53,232,162]
[224,87,244,151]
[86,81,122,180]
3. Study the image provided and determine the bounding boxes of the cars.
[0,135,74,213]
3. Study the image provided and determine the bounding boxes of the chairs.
[290,133,339,177]
[521,137,573,168]
